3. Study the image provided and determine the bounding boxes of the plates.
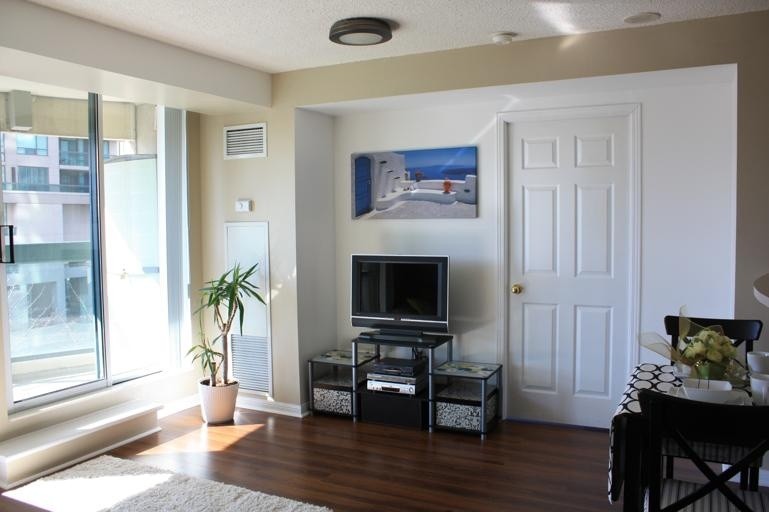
[676,387,745,407]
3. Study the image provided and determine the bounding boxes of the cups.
[749,374,769,406]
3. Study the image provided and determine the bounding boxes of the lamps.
[328,17,392,47]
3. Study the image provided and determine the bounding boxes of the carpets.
[1,451,333,512]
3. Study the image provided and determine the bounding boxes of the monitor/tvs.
[352,254,450,333]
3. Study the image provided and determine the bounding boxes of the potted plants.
[183,259,268,425]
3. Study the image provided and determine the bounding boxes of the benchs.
[0,398,168,491]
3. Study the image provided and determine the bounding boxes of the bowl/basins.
[746,351,769,374]
[682,378,732,404]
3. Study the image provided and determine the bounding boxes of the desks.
[305,331,504,443]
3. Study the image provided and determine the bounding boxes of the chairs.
[632,314,767,512]
